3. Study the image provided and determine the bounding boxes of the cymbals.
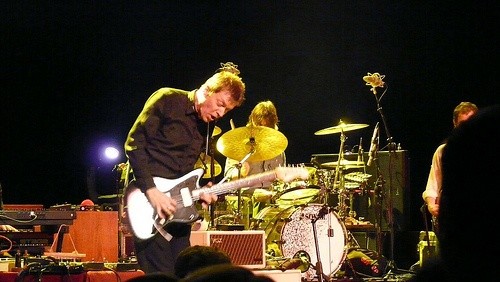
[216,127,289,162]
[193,153,222,179]
[315,125,371,133]
[321,159,367,171]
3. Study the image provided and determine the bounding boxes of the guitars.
[121,169,278,240]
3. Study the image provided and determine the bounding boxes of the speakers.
[189,231,265,270]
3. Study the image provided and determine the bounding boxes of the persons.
[124,71,286,282]
[405,101,500,282]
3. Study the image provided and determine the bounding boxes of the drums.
[275,184,322,204]
[269,201,348,278]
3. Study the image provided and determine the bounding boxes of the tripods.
[372,87,419,281]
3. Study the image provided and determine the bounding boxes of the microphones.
[358,138,362,161]
[366,138,378,166]
[368,123,379,156]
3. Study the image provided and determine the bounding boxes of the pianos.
[0,202,75,232]
